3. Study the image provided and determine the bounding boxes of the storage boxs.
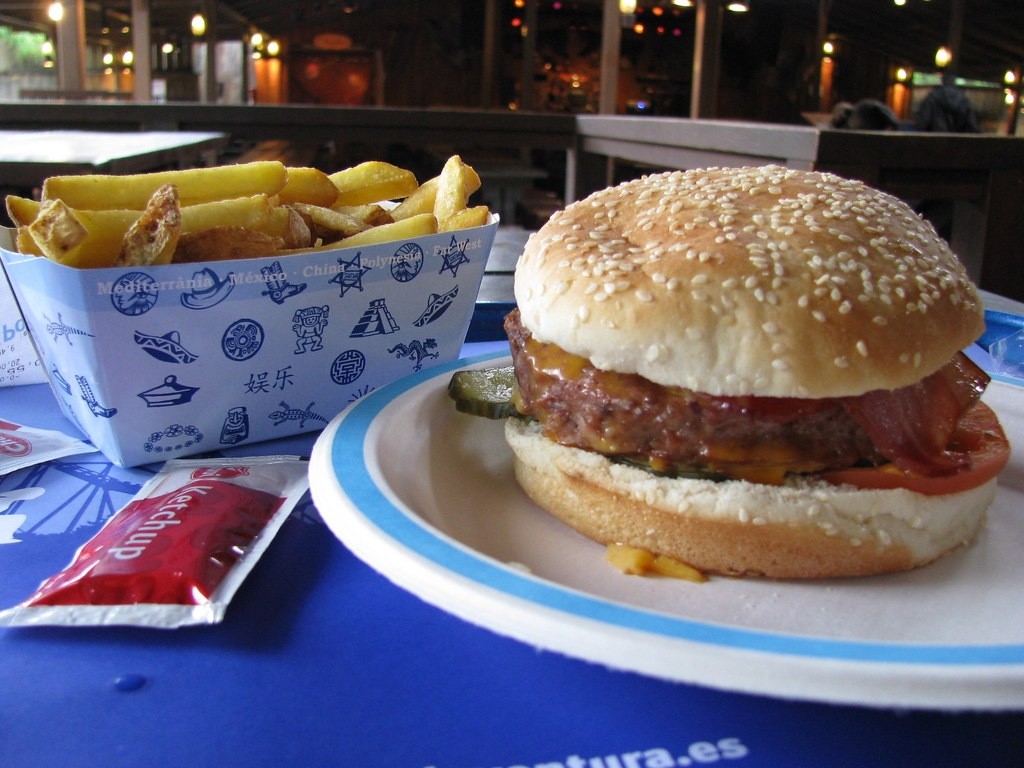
[1,199,500,468]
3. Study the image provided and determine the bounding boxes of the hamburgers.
[449,164,1012,581]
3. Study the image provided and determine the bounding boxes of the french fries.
[5,154,491,267]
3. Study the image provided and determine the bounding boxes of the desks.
[0,105,1024,768]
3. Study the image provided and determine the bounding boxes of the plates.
[307,350,1024,714]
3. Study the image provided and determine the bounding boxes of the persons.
[833,88,981,133]
